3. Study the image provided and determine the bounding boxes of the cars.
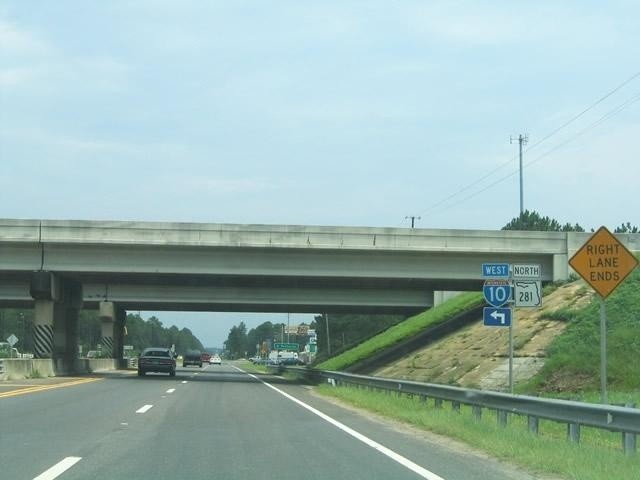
[249,356,305,366]
[183,349,221,368]
[138,347,178,376]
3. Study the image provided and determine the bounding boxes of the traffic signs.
[274,343,299,350]
[483,263,542,308]
